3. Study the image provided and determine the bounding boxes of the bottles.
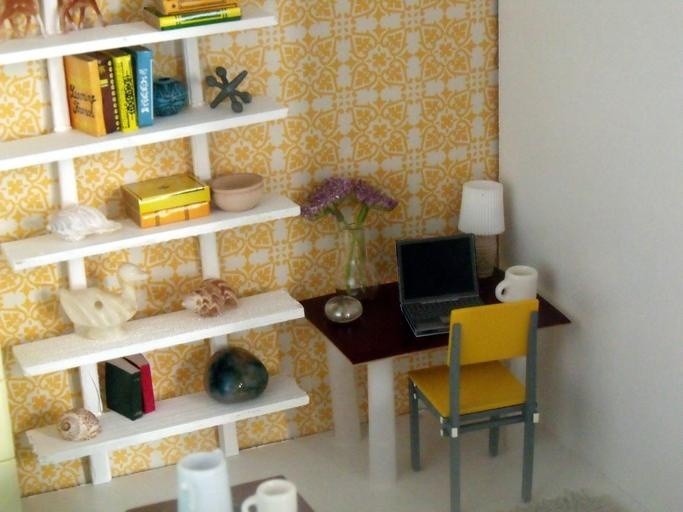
[153,76,186,116]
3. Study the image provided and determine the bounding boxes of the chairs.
[407,298,541,512]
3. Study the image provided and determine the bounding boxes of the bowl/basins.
[208,172,266,212]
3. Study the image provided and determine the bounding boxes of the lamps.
[456,180,510,289]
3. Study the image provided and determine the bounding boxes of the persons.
[63,45,155,138]
[105,353,155,421]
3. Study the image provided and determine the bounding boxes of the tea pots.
[176,446,229,511]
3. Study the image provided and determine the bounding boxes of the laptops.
[395,232,489,338]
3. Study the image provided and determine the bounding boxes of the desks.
[299,268,571,484]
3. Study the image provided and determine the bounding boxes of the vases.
[333,223,382,303]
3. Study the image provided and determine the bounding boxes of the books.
[144,0,241,31]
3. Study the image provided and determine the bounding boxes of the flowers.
[299,175,399,296]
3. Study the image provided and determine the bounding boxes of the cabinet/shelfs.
[1,0,309,484]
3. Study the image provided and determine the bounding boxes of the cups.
[240,481,300,510]
[497,263,541,310]
